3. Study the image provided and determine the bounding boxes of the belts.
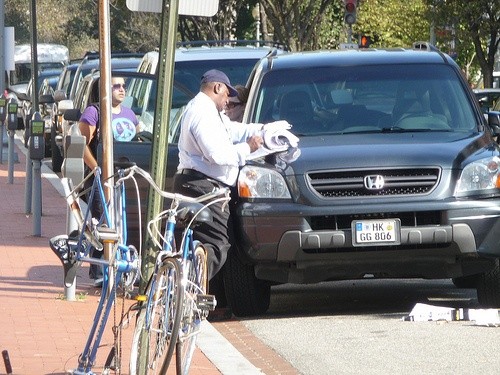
[175,168,229,189]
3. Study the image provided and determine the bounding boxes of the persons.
[78,74,142,287]
[171,69,264,282]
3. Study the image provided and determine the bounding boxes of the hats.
[200,68,239,97]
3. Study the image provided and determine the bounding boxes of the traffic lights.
[344,0,357,23]
[360,36,368,46]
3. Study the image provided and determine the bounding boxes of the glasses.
[113,83,128,90]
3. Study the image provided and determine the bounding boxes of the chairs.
[392,87,434,128]
[277,91,324,133]
[173,73,194,98]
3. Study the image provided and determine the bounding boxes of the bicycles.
[114,165,231,375]
[48,165,146,375]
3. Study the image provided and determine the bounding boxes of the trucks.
[6,45,70,129]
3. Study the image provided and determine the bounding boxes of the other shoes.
[95,278,103,287]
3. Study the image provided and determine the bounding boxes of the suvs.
[25,50,148,172]
[123,43,291,146]
[95,42,500,316]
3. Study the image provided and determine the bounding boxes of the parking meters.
[0,95,7,165]
[29,112,46,236]
[8,98,18,183]
[25,108,33,214]
